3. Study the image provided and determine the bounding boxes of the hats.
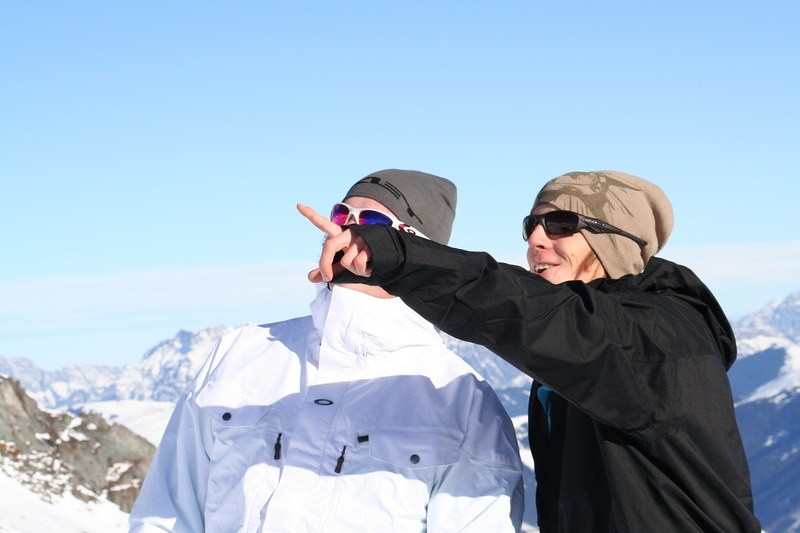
[341,169,457,245]
[531,171,674,278]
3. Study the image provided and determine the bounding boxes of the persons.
[128,168,524,533]
[297,171,763,533]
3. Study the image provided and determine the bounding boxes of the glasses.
[330,203,430,240]
[523,210,648,246]
